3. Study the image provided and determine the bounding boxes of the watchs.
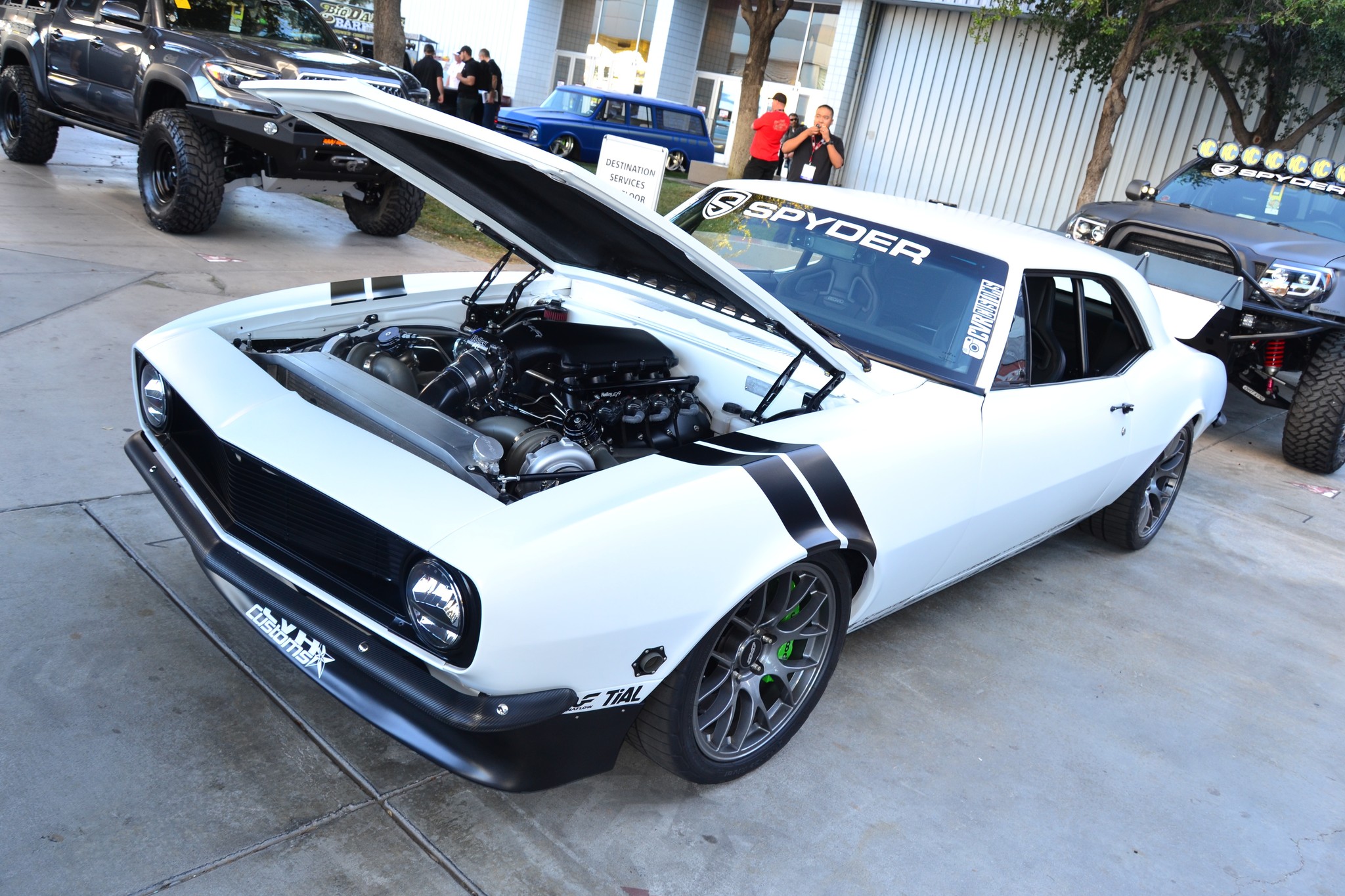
[825,139,834,146]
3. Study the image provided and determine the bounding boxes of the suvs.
[1056,139,1344,471]
[2,0,447,240]
[498,83,715,173]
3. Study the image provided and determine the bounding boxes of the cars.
[127,80,1228,804]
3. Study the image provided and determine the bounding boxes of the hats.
[457,46,471,55]
[769,93,786,103]
[424,44,434,52]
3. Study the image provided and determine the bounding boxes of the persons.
[782,112,810,180]
[781,104,845,186]
[455,45,482,123]
[411,44,444,111]
[445,51,466,90]
[479,48,503,131]
[741,92,791,181]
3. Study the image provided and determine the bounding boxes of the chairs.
[1024,275,1068,382]
[775,246,881,326]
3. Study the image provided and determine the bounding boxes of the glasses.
[790,117,798,121]
